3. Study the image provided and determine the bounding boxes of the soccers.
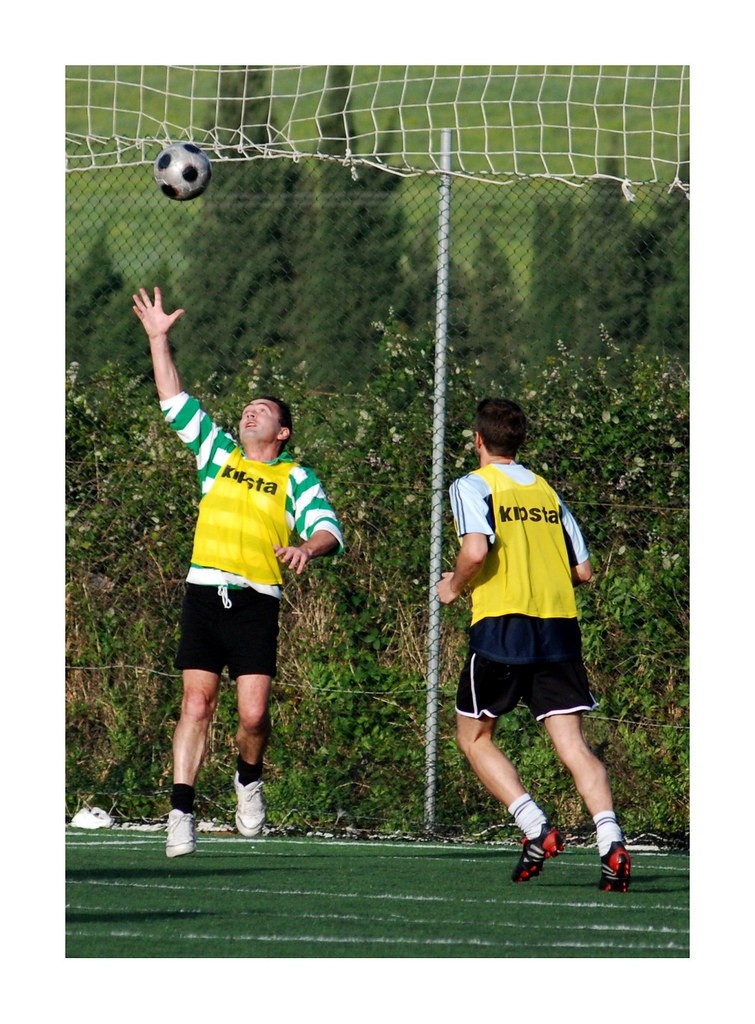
[154,140,212,201]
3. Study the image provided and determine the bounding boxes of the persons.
[437,398,631,891]
[132,287,345,857]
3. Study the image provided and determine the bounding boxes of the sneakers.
[512,819,564,882]
[164,809,197,857]
[599,841,631,892]
[234,770,266,837]
